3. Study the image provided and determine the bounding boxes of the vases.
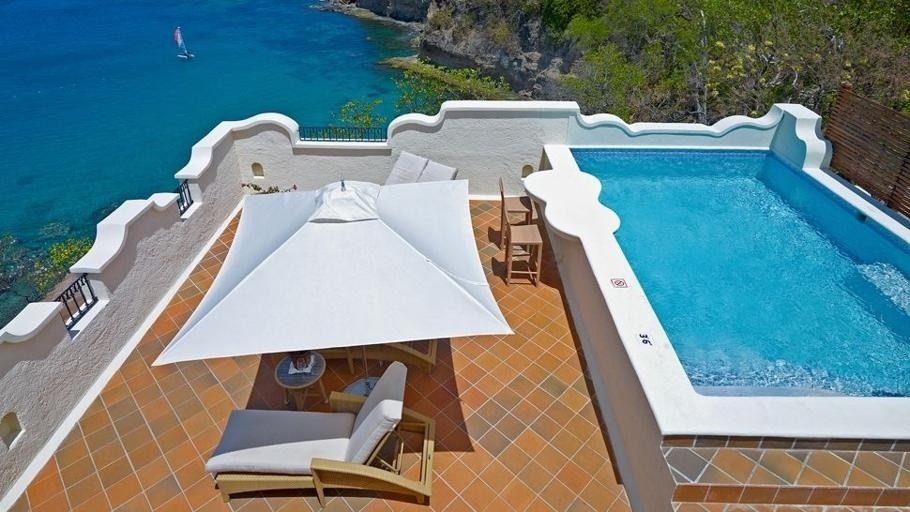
[288,350,312,371]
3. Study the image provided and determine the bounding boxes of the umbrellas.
[149,175,516,373]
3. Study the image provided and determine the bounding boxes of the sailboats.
[173,25,196,60]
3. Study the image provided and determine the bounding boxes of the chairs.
[383,151,459,187]
[203,361,437,506]
[499,178,544,287]
